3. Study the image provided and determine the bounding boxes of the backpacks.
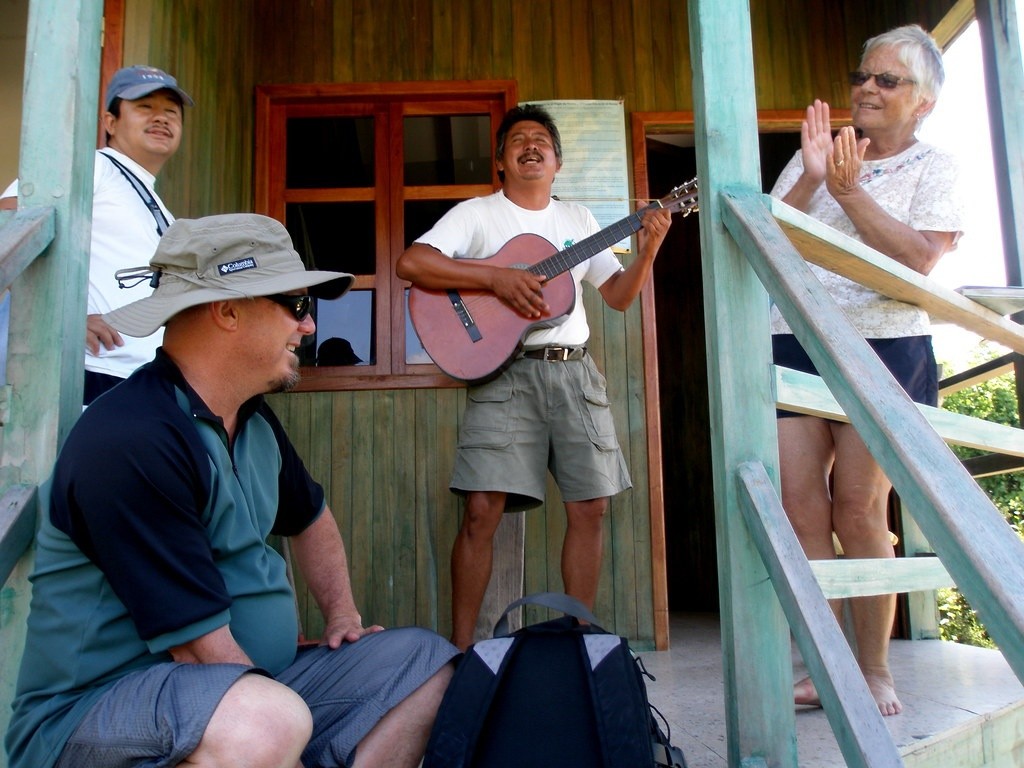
[422,587,690,768]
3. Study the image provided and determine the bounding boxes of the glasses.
[260,293,313,322]
[847,70,917,89]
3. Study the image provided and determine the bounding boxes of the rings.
[836,159,843,166]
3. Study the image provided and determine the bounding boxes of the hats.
[104,64,194,110]
[100,212,355,338]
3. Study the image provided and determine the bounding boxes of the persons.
[0,64,195,404]
[395,100,673,653]
[2,212,463,768]
[768,24,963,715]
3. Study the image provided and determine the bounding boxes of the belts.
[523,347,587,362]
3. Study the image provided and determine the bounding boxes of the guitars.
[406,173,701,391]
